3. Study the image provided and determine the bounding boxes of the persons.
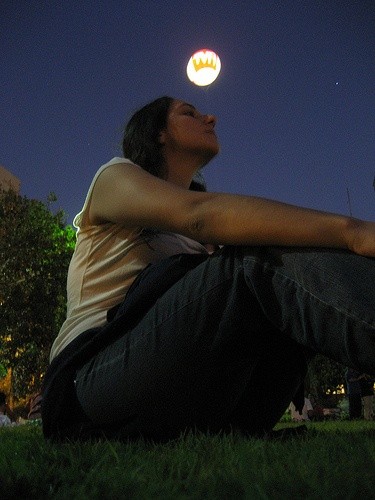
[39,94,375,433]
[289,393,315,423]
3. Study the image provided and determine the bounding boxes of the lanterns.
[186,48,222,86]
